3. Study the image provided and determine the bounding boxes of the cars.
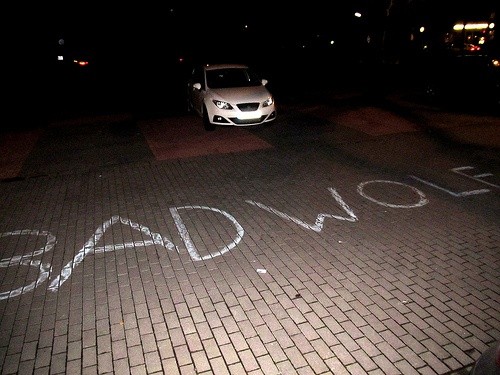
[185,63,279,129]
